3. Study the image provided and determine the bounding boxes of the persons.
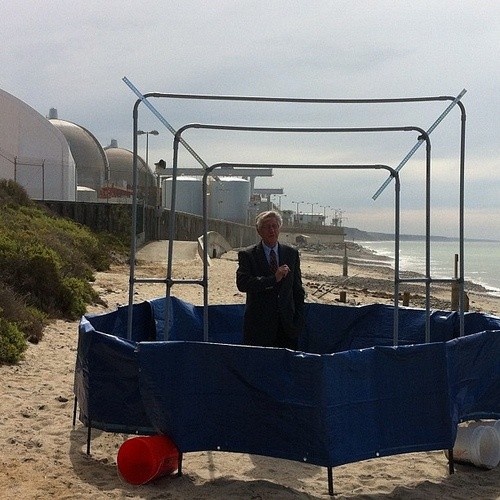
[236,211,305,349]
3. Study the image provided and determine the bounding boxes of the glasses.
[261,223,280,230]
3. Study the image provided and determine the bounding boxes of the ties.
[270,249,278,307]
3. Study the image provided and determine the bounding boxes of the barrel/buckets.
[117,435,179,487]
[443,425,500,470]
[469,420,500,427]
